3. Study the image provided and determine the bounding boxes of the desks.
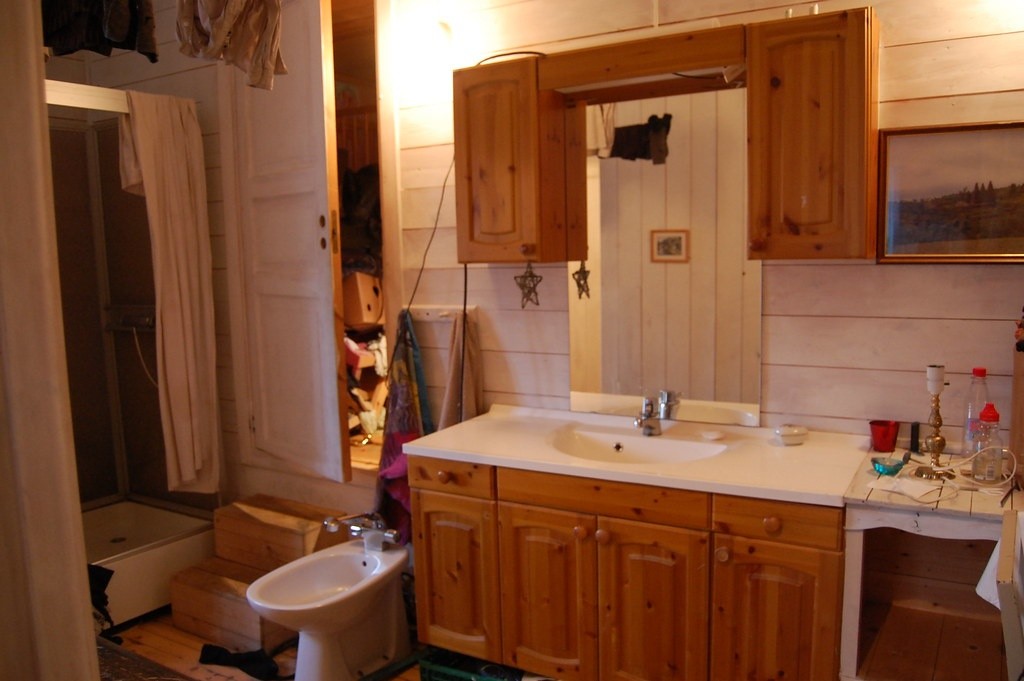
[838,438,1023,681]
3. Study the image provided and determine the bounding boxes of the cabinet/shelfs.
[406,455,845,681]
[745,6,878,257]
[453,56,568,262]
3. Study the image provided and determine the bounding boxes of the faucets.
[633,398,662,436]
[658,389,680,422]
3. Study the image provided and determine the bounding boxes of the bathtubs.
[81,491,216,632]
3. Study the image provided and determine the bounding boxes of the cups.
[869,419,899,452]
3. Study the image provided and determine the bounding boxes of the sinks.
[548,422,738,467]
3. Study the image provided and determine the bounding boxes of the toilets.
[245,538,412,681]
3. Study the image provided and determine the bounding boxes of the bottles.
[962,367,990,457]
[971,402,1003,483]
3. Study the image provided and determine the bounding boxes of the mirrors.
[568,71,762,428]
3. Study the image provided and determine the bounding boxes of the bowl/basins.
[872,458,904,476]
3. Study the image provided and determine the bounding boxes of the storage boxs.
[343,272,384,331]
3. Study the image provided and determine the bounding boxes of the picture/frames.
[876,121,1024,264]
[650,230,690,263]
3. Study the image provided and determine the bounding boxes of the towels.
[375,308,425,549]
[438,310,485,432]
